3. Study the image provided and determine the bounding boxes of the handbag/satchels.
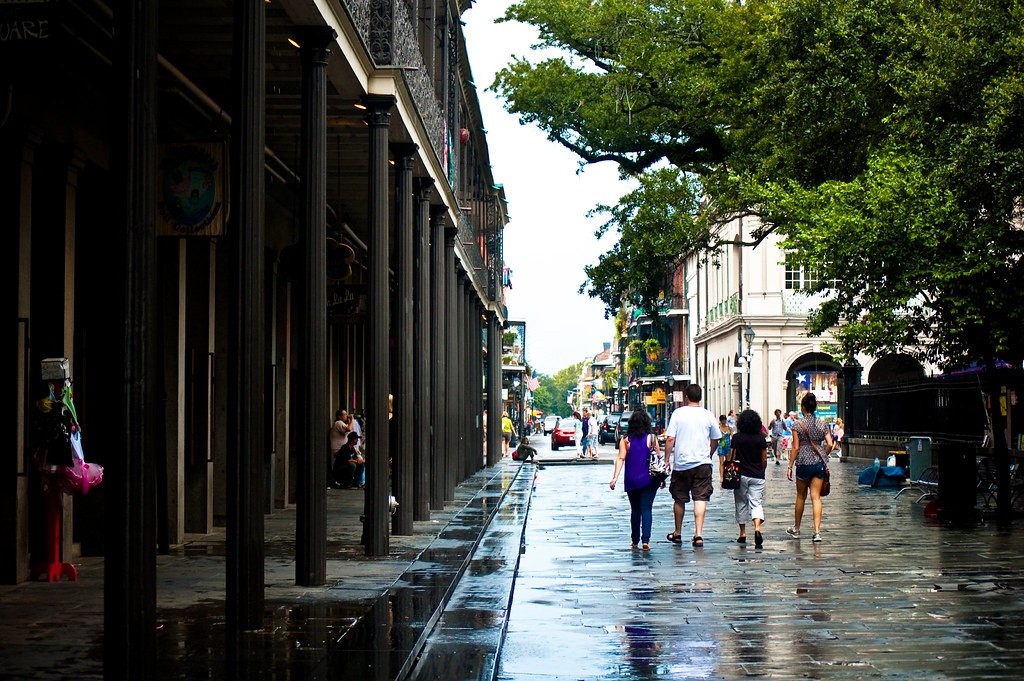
[721,461,741,488]
[513,451,519,460]
[819,469,830,496]
[649,434,670,482]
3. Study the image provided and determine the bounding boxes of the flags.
[592,387,606,404]
[567,391,573,403]
[528,369,540,391]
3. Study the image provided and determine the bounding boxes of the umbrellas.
[939,355,1014,439]
[533,410,543,416]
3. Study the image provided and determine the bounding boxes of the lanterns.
[460,128,469,144]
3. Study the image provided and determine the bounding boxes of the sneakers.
[811,532,822,541]
[785,526,800,539]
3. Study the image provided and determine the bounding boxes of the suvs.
[597,411,656,449]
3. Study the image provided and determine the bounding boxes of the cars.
[551,419,582,450]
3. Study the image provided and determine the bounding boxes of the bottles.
[873,457,879,466]
[887,454,896,467]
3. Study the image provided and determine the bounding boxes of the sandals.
[667,533,682,543]
[632,542,638,550]
[643,543,650,551]
[693,535,704,547]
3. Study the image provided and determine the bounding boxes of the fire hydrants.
[359,482,399,545]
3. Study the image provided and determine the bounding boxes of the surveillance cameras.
[738,357,746,364]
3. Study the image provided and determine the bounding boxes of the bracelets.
[788,465,793,470]
[613,478,618,481]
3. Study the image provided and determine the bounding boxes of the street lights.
[744,322,756,409]
[665,371,677,413]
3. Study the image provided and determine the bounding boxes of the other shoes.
[353,482,362,487]
[776,461,779,465]
[755,531,763,544]
[737,537,746,541]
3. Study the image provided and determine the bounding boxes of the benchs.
[895,458,1024,516]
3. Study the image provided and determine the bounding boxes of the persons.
[330,410,368,490]
[786,393,833,541]
[573,409,599,459]
[516,438,538,464]
[828,418,844,459]
[610,411,666,550]
[729,410,768,545]
[556,417,559,427]
[483,410,486,444]
[500,411,516,457]
[718,410,736,483]
[664,384,722,546]
[763,409,800,465]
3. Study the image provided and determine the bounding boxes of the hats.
[348,432,363,440]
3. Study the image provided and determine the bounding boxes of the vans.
[541,416,563,436]
